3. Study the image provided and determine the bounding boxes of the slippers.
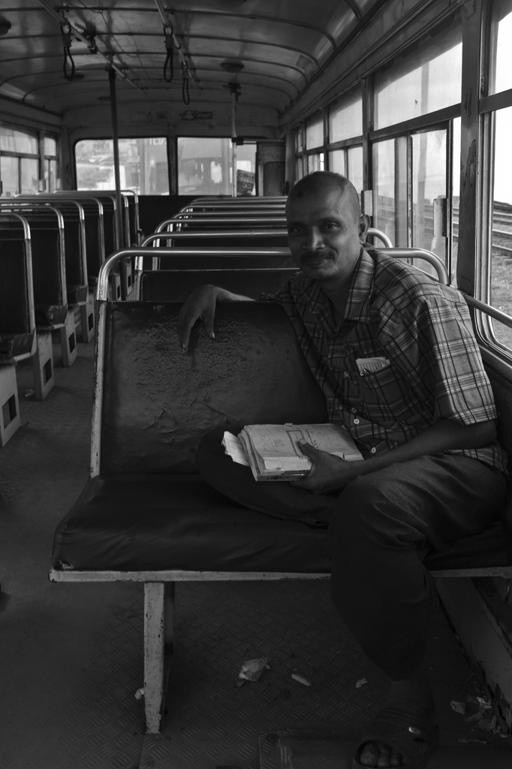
[355,697,439,767]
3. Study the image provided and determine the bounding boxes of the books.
[222,419,365,482]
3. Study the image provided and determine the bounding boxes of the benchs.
[0,191,143,448]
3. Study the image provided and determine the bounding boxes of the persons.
[174,170,510,769]
